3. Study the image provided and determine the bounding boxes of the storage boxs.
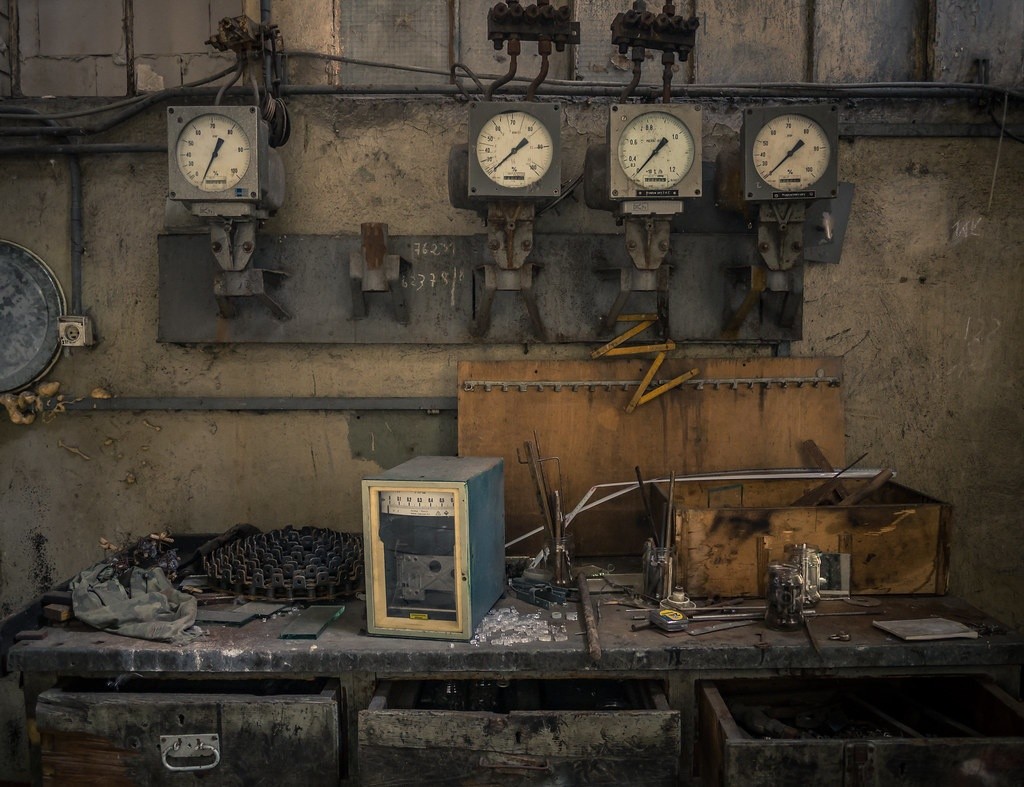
[653,473,953,600]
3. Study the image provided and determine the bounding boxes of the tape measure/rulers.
[649,608,689,632]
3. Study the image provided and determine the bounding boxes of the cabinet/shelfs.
[10,548,1023,787]
